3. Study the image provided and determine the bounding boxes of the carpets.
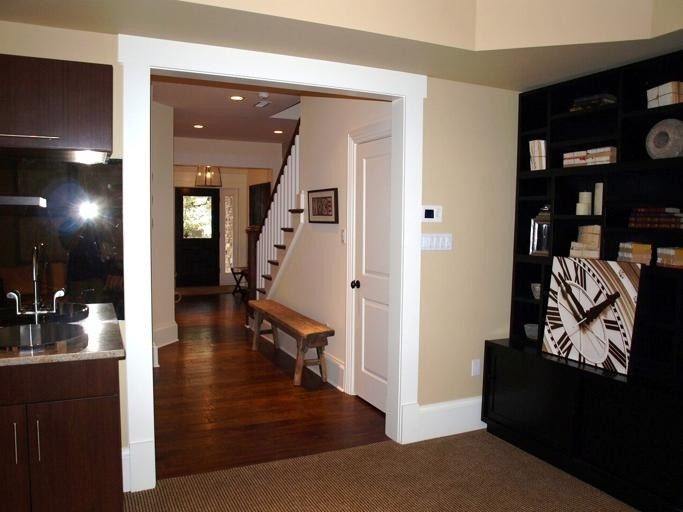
[123,428,635,511]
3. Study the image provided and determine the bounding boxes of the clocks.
[537,258,641,375]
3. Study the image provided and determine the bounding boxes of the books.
[648,82,683,106]
[564,147,617,166]
[570,225,600,259]
[628,207,683,228]
[529,139,547,170]
[617,242,683,266]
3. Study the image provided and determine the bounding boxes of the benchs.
[248,300,335,386]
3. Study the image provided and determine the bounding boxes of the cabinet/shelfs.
[0,53,123,301]
[0,302,126,512]
[483,49,683,512]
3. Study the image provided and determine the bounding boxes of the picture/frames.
[247,182,272,227]
[308,188,339,224]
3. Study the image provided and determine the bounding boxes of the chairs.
[226,267,251,298]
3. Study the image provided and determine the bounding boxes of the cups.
[524,324,538,339]
[531,283,541,299]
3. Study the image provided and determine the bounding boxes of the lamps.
[195,165,222,187]
